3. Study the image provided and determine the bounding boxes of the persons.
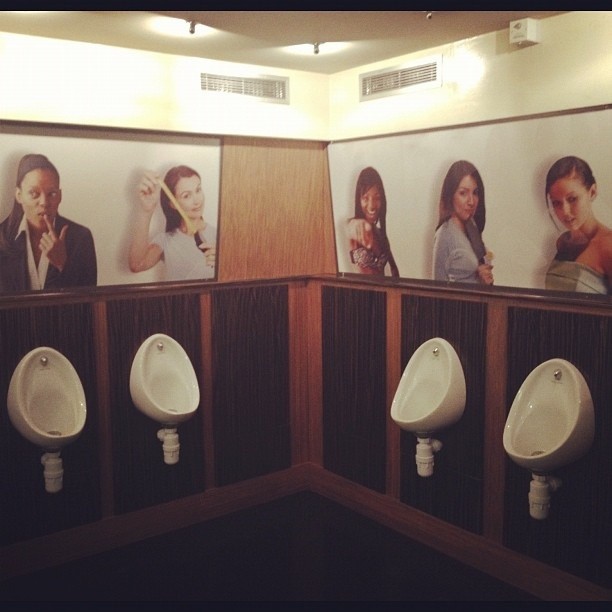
[345,167,399,276]
[545,156,612,294]
[128,165,217,280]
[1,153,97,292]
[433,160,494,284]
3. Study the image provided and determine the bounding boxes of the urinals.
[503,357,596,521]
[389,338,467,477]
[128,334,200,464]
[4,347,86,495]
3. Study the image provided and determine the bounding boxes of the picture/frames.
[1,118,224,301]
[326,104,611,306]
